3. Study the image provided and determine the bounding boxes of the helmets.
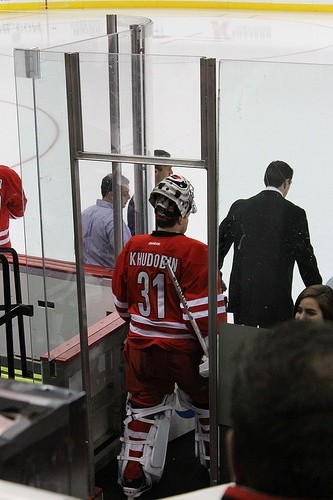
[149,174,196,219]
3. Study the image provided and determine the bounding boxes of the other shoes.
[120,478,149,500]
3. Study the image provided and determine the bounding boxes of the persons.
[80,173,132,269]
[292,285,333,322]
[0,165,27,250]
[217,160,322,329]
[110,175,225,500]
[128,150,173,238]
[220,318,333,500]
